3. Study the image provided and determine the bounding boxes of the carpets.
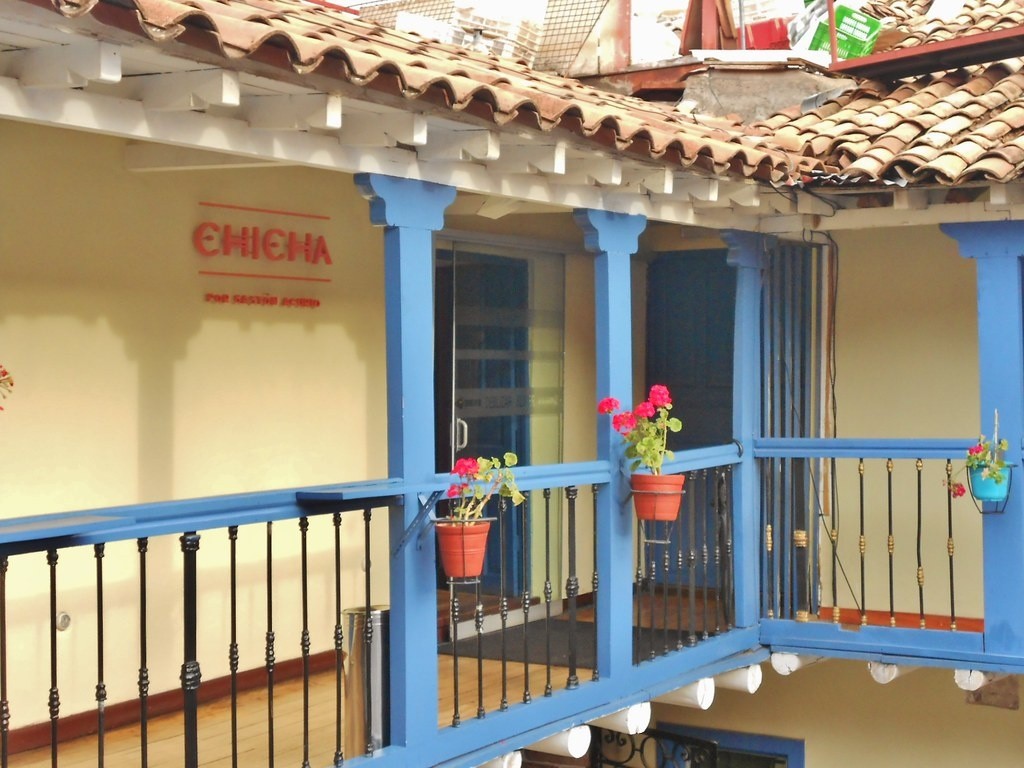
[437,616,716,668]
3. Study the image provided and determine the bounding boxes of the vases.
[969,462,1014,502]
[435,522,490,578]
[631,474,685,521]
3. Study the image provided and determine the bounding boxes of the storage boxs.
[810,6,881,60]
[734,19,791,51]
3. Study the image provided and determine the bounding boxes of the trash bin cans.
[342,605,391,760]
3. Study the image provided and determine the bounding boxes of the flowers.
[943,437,1008,497]
[446,453,525,525]
[597,384,682,475]
